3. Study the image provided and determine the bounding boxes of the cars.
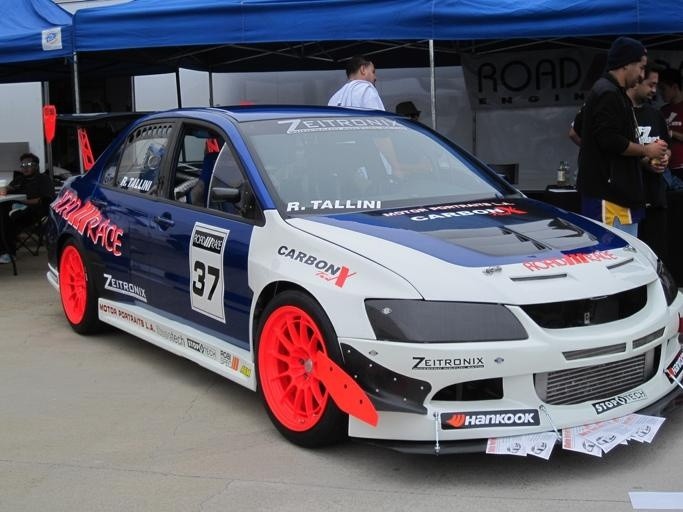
[42,104,682,457]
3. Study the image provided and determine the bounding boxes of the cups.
[0,180,6,195]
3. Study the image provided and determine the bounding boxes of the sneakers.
[9,204,29,218]
[0,253,16,263]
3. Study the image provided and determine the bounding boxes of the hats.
[608,37,647,68]
[396,101,421,116]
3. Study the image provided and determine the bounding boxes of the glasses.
[21,161,36,168]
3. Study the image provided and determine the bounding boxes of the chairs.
[11,169,49,256]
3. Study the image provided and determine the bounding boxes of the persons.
[1,152,45,263]
[569,36,683,237]
[395,101,421,121]
[328,55,386,111]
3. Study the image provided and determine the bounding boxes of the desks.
[522,189,581,212]
[0,194,25,276]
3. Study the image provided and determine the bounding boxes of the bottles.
[648,138,664,169]
[556,160,565,184]
[564,160,570,185]
